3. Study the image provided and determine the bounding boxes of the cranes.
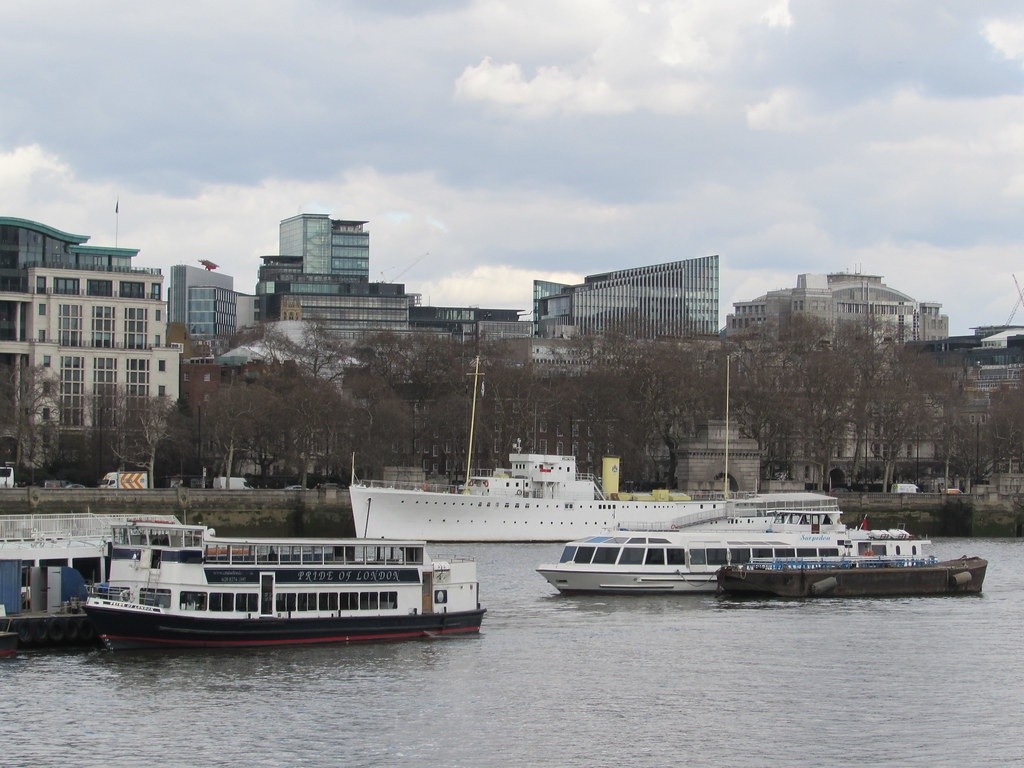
[1003,274,1024,325]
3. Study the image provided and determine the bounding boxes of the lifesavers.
[517,489,522,495]
[671,524,676,529]
[15,618,96,645]
[484,480,489,487]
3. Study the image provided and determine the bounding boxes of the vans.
[891,484,920,493]
[213,477,254,490]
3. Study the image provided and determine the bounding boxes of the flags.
[862,518,867,530]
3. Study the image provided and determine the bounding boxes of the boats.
[716,555,988,595]
[347,453,842,542]
[535,497,939,595]
[83,516,487,643]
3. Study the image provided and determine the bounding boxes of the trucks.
[99,471,148,489]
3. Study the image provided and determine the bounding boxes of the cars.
[284,484,310,490]
[65,485,85,489]
